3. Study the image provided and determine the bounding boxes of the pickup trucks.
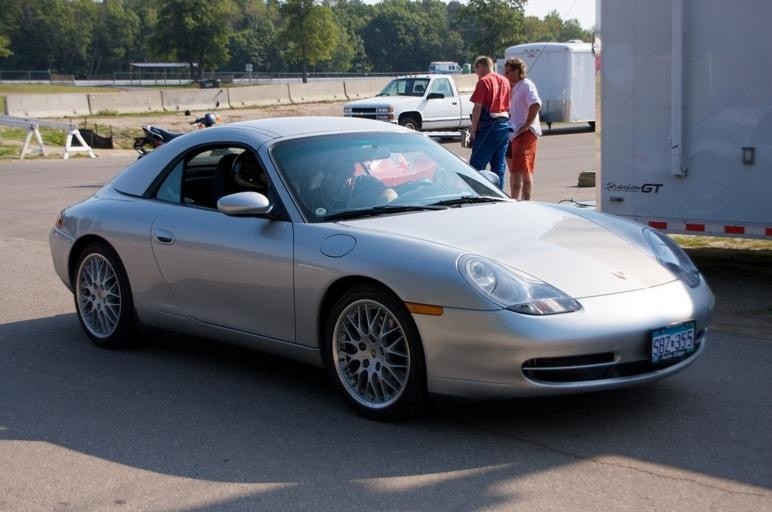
[343,71,500,132]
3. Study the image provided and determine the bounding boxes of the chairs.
[415,84,425,97]
[181,151,326,214]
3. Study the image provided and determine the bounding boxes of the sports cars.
[49,115,716,416]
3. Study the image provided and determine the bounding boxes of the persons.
[287,146,325,196]
[504,57,542,200]
[308,160,399,209]
[467,56,512,192]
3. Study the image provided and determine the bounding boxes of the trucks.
[598,2,769,237]
[504,37,598,131]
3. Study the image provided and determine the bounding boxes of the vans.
[429,62,461,74]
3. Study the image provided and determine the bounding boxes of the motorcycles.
[133,101,220,158]
